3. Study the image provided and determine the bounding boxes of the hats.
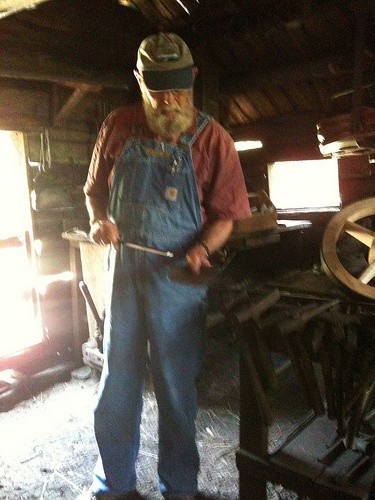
[136,31,196,91]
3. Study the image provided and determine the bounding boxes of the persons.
[82,32,253,500]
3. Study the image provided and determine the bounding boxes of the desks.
[233,270,375,500]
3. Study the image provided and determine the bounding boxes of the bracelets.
[196,241,214,259]
